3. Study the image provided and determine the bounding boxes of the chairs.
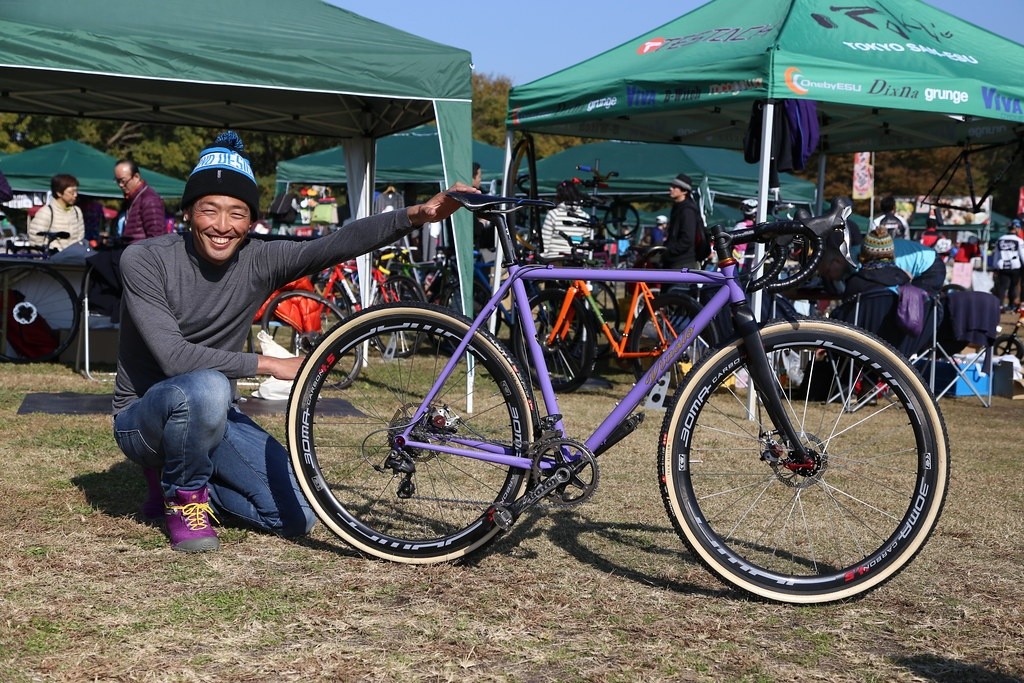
[917,284,1002,380]
[794,286,944,410]
[74,243,130,384]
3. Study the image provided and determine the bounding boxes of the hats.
[180,130,260,223]
[861,225,895,261]
[671,174,692,193]
[1012,219,1021,226]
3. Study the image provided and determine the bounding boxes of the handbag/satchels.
[311,200,340,225]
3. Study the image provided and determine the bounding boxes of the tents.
[2,0,1024,417]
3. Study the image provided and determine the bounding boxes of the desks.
[0,256,120,367]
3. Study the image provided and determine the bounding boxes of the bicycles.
[994,304,1024,363]
[262,164,718,408]
[284,189,951,606]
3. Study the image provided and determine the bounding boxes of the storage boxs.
[992,361,1014,400]
[915,354,994,396]
[959,342,983,354]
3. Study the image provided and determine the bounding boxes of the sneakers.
[144,462,165,520]
[162,482,220,553]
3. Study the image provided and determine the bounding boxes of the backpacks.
[270,192,299,222]
[678,199,711,261]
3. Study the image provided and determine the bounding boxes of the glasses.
[112,175,134,190]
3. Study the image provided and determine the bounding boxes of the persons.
[2,132,1024,552]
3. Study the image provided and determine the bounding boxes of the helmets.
[740,198,758,215]
[656,215,668,225]
[935,238,952,253]
